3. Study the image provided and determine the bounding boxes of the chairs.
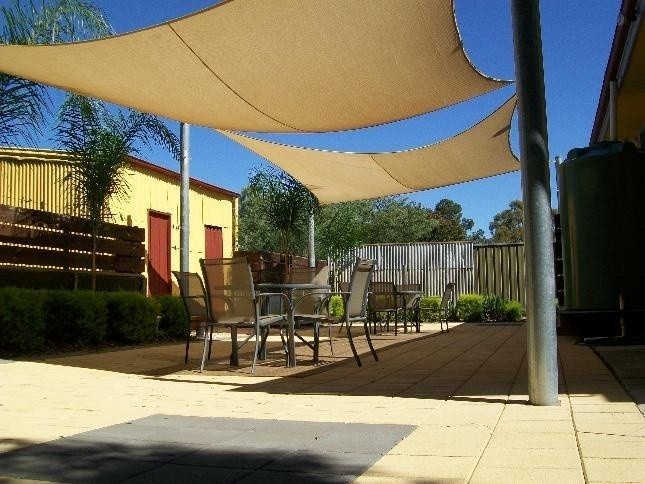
[170,255,456,374]
